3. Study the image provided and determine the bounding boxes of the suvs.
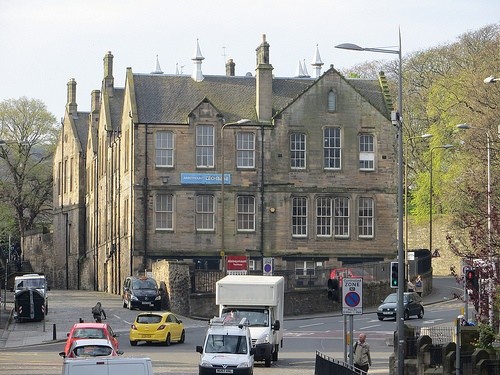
[196,324,255,375]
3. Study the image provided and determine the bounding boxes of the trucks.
[215,274,285,368]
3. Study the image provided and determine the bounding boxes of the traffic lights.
[465,270,473,289]
[390,262,399,288]
[327,279,338,301]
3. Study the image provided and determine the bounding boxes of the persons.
[224,309,240,323]
[408,276,423,297]
[348,334,372,375]
[91,301,107,323]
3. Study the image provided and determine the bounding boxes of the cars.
[65,322,119,356]
[129,311,185,346]
[377,292,425,321]
[60,338,124,360]
[122,275,162,310]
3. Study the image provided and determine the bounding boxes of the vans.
[13,275,48,303]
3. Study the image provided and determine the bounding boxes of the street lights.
[219,118,252,253]
[334,29,407,375]
[429,144,451,251]
[406,134,433,261]
[456,122,495,328]
